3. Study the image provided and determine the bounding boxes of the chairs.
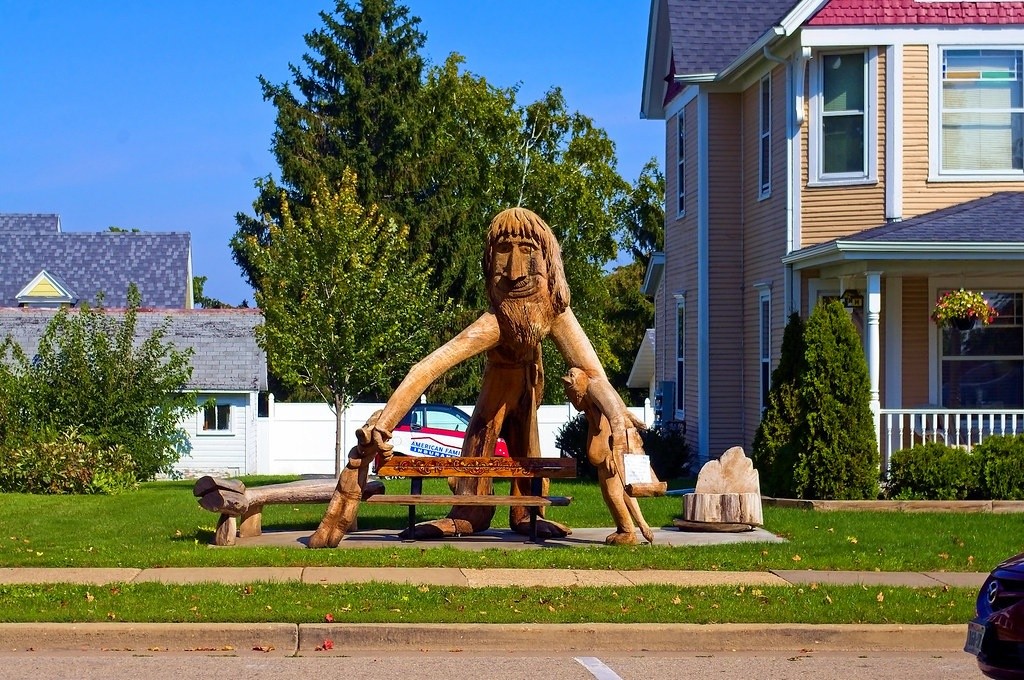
[673,446,763,532]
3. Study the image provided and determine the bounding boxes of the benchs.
[359,456,578,543]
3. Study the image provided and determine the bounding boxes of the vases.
[951,316,977,332]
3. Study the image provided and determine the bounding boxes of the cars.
[963,551,1024,679]
[373,401,511,461]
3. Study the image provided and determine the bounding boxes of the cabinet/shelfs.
[654,381,675,431]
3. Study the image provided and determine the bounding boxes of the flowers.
[931,286,998,332]
